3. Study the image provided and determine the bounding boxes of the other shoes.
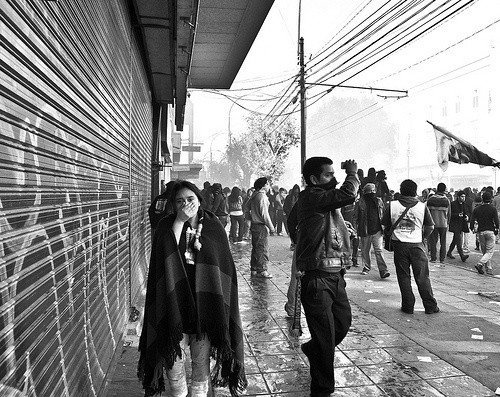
[362,267,369,274]
[475,264,484,273]
[462,255,469,262]
[425,307,439,314]
[447,254,455,259]
[251,269,272,278]
[380,270,390,278]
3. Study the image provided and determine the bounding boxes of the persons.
[137,181,247,397]
[379,179,440,314]
[417,185,500,255]
[470,192,499,275]
[286,157,361,397]
[146,181,177,231]
[447,190,469,262]
[194,177,301,279]
[339,167,399,278]
[427,183,451,263]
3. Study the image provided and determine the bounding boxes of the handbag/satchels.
[383,234,393,251]
